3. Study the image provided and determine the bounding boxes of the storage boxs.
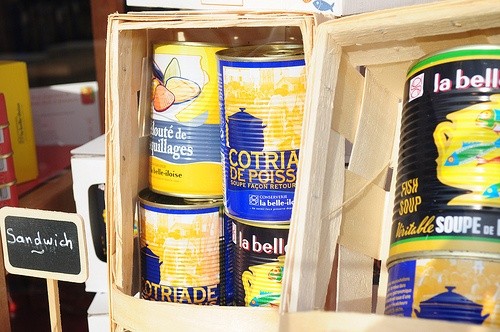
[0,0,500,332]
